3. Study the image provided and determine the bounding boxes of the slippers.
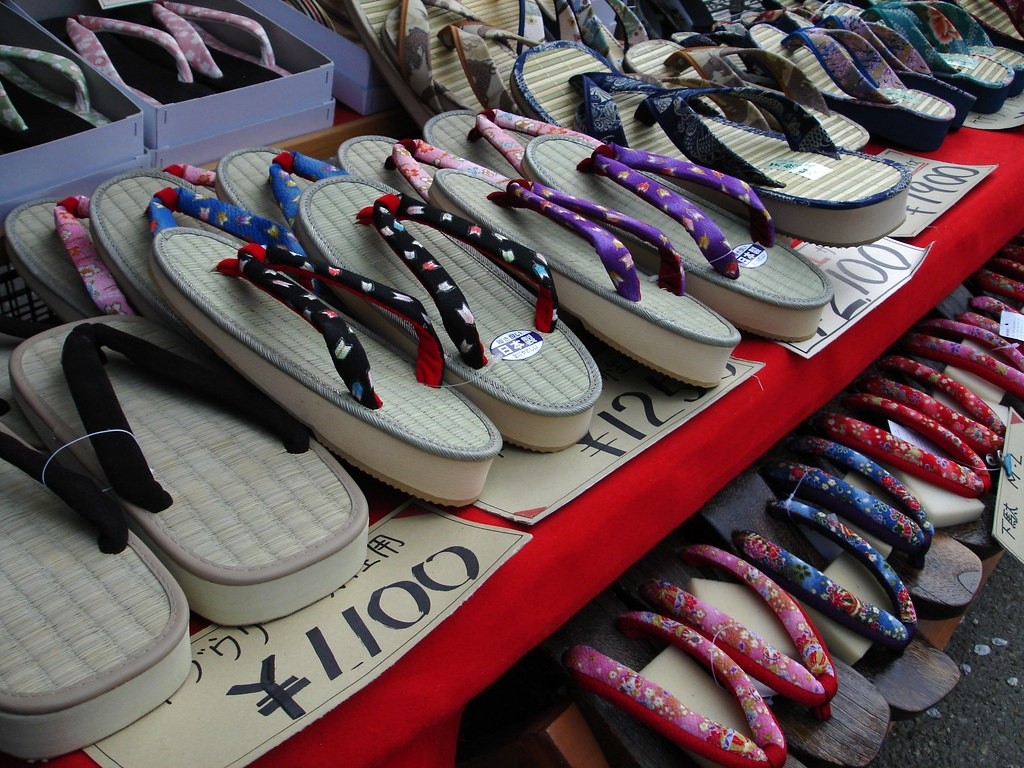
[147,227,503,507]
[427,167,742,388]
[519,134,834,341]
[0,0,1024,318]
[554,230,1024,768]
[8,314,369,626]
[299,176,603,454]
[0,423,192,759]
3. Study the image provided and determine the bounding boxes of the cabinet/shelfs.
[0,0,1024,768]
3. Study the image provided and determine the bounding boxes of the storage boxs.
[0,0,410,220]
